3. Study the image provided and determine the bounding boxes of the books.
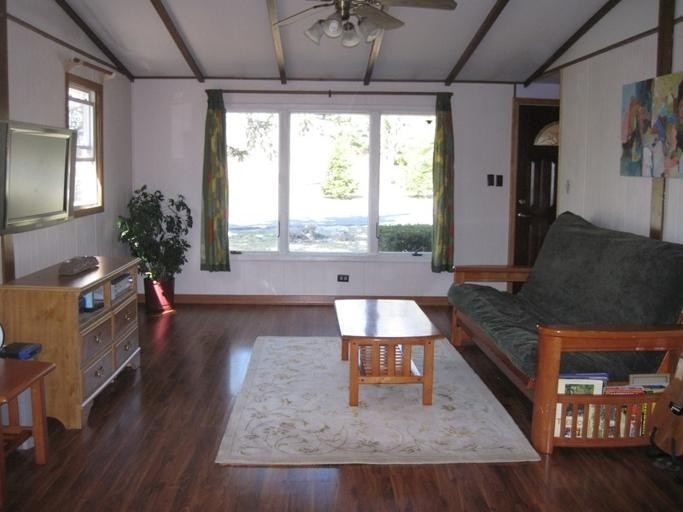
[552,372,671,438]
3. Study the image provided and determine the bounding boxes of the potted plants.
[114,185,193,315]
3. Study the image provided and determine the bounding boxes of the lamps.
[304,0,381,48]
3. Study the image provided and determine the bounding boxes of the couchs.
[448,212,683,456]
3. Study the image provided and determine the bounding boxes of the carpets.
[211,335,540,465]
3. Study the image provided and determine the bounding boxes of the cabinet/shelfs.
[0,257,140,431]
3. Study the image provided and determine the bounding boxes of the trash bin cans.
[0,325,41,450]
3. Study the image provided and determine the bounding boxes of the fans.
[273,1,458,35]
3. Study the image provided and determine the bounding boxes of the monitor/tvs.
[0,120,77,235]
